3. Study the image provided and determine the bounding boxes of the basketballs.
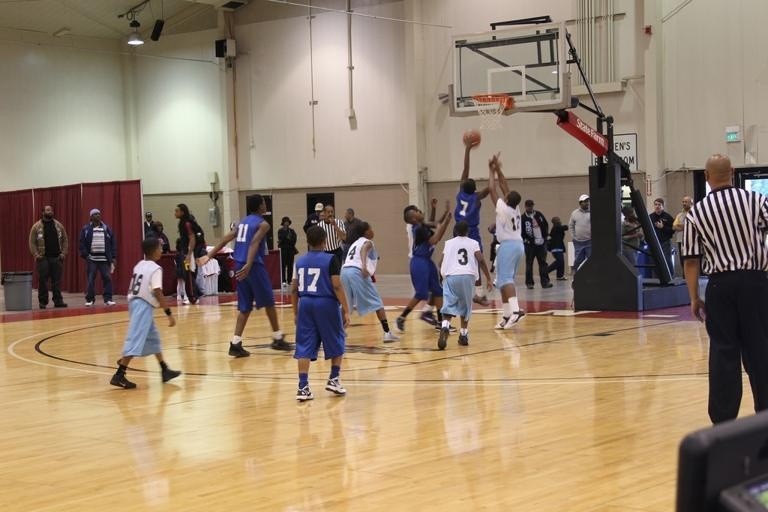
[463,130,481,149]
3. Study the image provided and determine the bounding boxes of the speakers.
[214,39,236,58]
[151,19,164,41]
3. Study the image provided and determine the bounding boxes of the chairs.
[677,408,767,509]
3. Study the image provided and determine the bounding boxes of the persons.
[396,209,456,332]
[141,133,695,308]
[437,220,494,350]
[488,157,526,331]
[340,222,400,343]
[109,237,183,389]
[403,199,450,326]
[292,225,349,401]
[27,202,71,310]
[195,194,292,356]
[682,154,768,424]
[76,206,120,307]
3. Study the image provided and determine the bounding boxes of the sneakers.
[229,341,249,356]
[110,374,136,388]
[384,311,467,349]
[472,294,488,305]
[543,283,553,288]
[296,386,314,400]
[495,317,509,328]
[162,369,181,382]
[183,299,199,304]
[527,284,534,289]
[504,311,526,329]
[325,376,346,394]
[272,335,291,350]
[39,301,117,308]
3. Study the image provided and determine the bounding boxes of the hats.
[90,209,100,216]
[315,203,323,210]
[579,194,590,201]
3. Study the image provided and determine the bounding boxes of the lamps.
[208,173,219,228]
[116,1,167,49]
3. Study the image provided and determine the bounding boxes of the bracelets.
[164,308,172,316]
